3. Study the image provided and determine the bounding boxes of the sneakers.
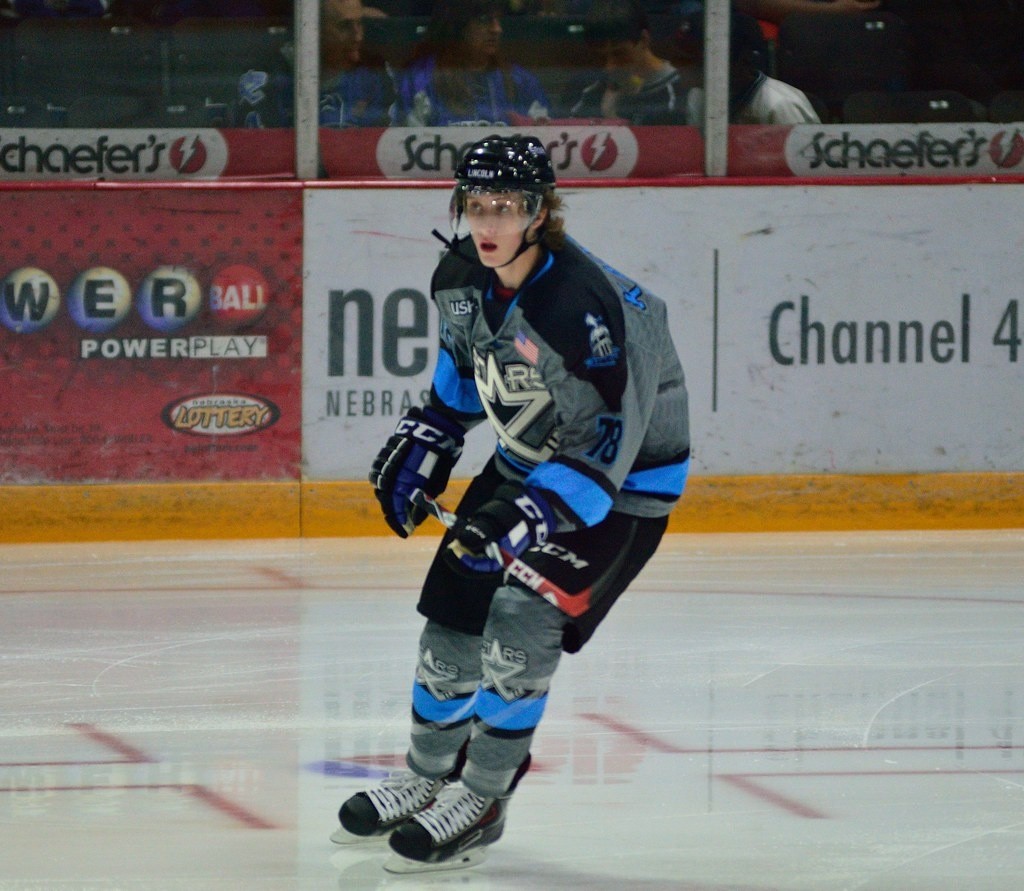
[383,778,507,874]
[329,766,459,847]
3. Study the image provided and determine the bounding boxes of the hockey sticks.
[408,486,644,621]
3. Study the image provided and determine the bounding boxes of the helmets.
[453,133,557,193]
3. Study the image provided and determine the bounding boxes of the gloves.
[368,406,468,539]
[444,479,589,587]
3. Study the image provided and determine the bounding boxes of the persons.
[333,137,687,874]
[226,0,917,129]
